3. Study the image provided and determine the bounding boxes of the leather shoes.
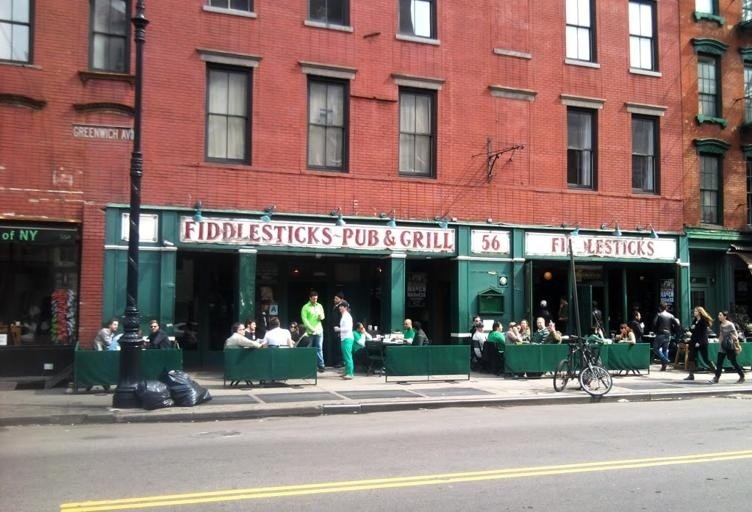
[661,360,670,370]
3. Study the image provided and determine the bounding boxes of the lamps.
[191,201,204,223]
[330,206,346,227]
[435,211,450,230]
[637,222,659,239]
[380,208,398,230]
[600,219,622,237]
[259,205,277,224]
[561,220,583,237]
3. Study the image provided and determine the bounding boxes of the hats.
[336,300,349,307]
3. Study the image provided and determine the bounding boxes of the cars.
[173,320,200,348]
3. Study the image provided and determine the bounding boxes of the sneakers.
[339,374,352,379]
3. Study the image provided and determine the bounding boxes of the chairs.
[364,340,386,377]
[618,368,640,376]
[469,340,486,370]
[673,342,690,371]
[230,379,254,387]
[483,341,506,376]
[170,339,178,348]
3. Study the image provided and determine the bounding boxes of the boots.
[708,360,725,377]
[684,361,694,380]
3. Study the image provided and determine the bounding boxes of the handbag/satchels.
[721,335,735,352]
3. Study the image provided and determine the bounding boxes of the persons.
[616,324,635,344]
[592,301,602,326]
[20,305,42,344]
[141,319,171,349]
[595,325,607,339]
[92,319,122,350]
[733,321,746,343]
[706,310,746,384]
[653,301,679,371]
[300,291,325,372]
[403,319,429,346]
[255,300,269,339]
[685,304,718,381]
[469,315,549,355]
[558,296,569,333]
[227,317,300,348]
[538,300,550,322]
[629,311,643,343]
[330,291,354,380]
[351,321,371,370]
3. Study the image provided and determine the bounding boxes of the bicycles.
[553,333,613,396]
